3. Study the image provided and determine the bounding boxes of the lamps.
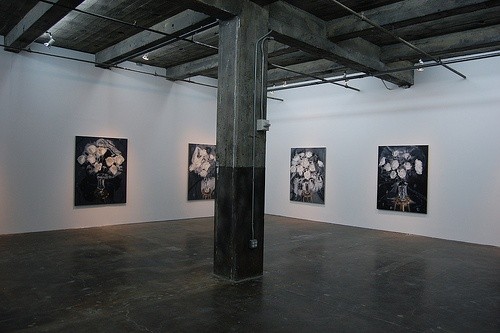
[43,32,55,50]
[416,57,424,72]
